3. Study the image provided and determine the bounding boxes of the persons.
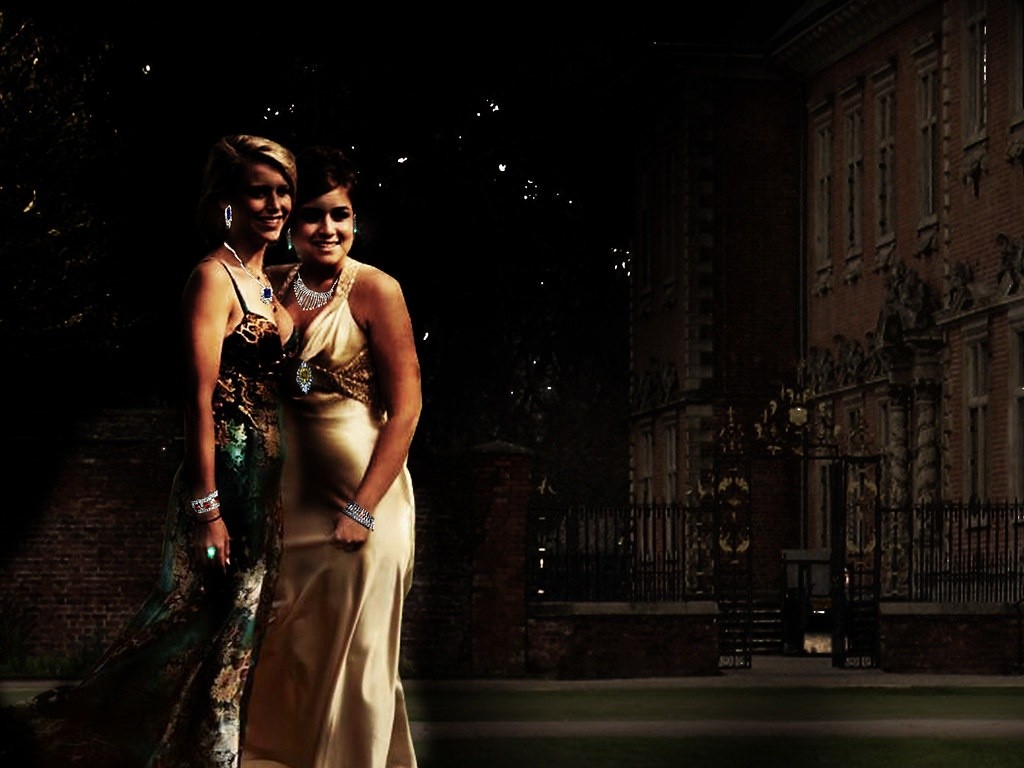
[240,147,422,768]
[154,133,299,768]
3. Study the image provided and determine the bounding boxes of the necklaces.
[223,240,273,304]
[293,270,339,311]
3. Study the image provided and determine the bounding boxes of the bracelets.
[339,500,374,531]
[182,489,222,525]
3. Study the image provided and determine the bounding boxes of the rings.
[204,545,216,560]
